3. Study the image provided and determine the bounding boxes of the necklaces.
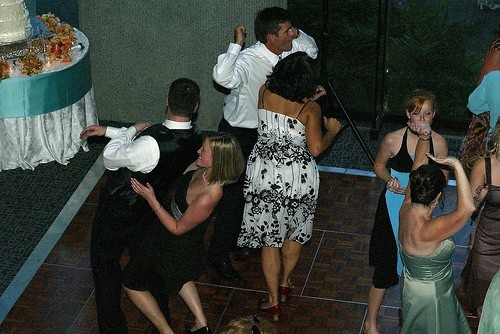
[408,126,420,137]
[202,170,226,186]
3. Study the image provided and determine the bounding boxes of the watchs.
[419,131,430,136]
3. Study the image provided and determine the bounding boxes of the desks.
[0,16,100,171]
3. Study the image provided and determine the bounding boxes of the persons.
[467,70,500,158]
[221,315,277,334]
[461,116,500,322]
[206,7,320,279]
[80,78,205,334]
[398,121,476,334]
[456,38,500,181]
[477,269,500,334]
[361,89,450,334]
[237,51,344,320]
[111,132,245,334]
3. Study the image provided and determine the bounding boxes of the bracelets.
[420,137,431,141]
[152,206,162,212]
[295,29,300,39]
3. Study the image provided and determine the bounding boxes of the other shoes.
[209,253,246,286]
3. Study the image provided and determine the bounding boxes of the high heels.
[258,298,280,322]
[278,286,291,302]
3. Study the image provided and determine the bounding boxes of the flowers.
[0,57,12,81]
[15,53,43,78]
[37,12,77,63]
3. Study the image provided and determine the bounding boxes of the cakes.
[0,0,30,42]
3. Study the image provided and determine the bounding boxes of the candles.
[9,68,14,75]
[44,60,53,69]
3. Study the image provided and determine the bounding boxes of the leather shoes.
[186,326,210,334]
